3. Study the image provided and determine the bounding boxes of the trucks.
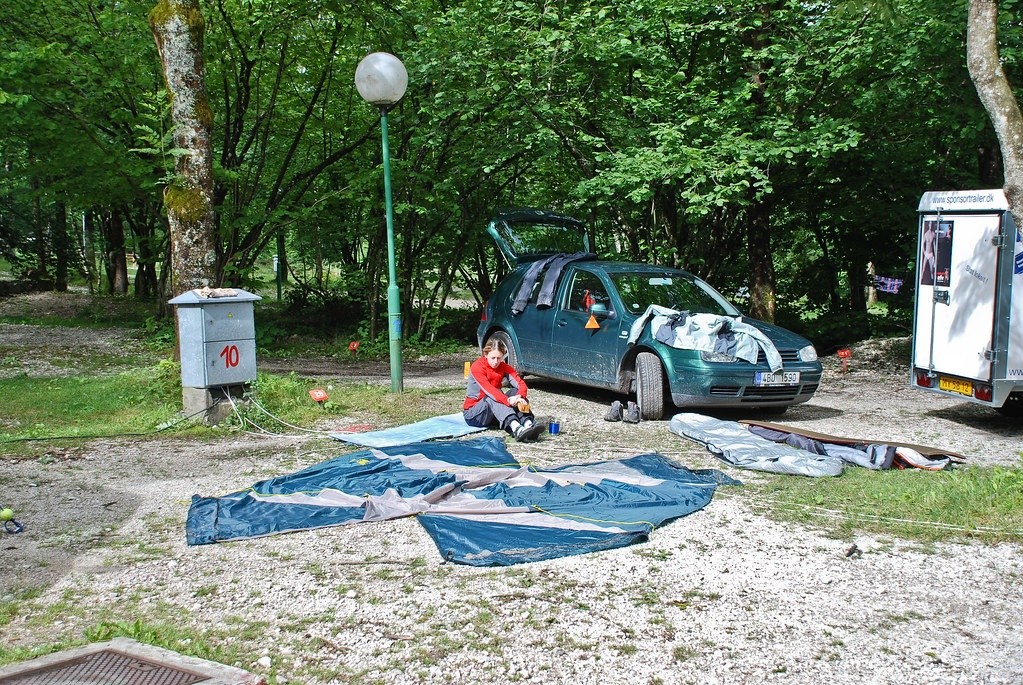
[910,186,1023,428]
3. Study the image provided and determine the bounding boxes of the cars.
[475,210,825,419]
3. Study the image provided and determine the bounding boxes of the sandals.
[514,424,546,442]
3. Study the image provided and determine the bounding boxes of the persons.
[464,337,546,444]
[922,221,935,284]
[946,225,952,239]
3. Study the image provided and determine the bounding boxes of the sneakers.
[603,401,623,422]
[623,401,641,424]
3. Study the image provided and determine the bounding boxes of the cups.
[549,423,559,435]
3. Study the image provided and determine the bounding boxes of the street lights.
[355,52,408,396]
